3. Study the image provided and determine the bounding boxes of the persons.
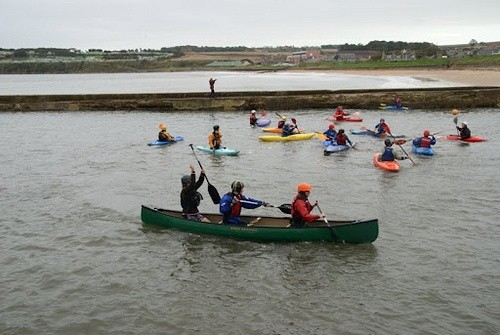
[281,121,298,137]
[277,116,287,129]
[158,124,175,141]
[322,124,337,141]
[381,138,408,162]
[220,181,273,226]
[375,119,387,134]
[333,129,352,148]
[180,164,211,222]
[334,106,350,121]
[208,125,226,152]
[456,121,471,140]
[209,76,218,98]
[290,184,325,226]
[417,130,436,148]
[250,110,258,126]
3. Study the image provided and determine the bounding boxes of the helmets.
[338,105,343,110]
[214,124,220,130]
[380,118,384,122]
[396,98,401,102]
[462,121,467,126]
[230,180,244,193]
[160,125,167,130]
[298,183,310,193]
[279,116,286,122]
[180,172,192,186]
[329,124,334,129]
[424,129,429,136]
[339,129,344,133]
[251,110,256,114]
[285,122,291,126]
[385,139,391,145]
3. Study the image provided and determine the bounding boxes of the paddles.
[313,130,360,152]
[359,127,387,138]
[315,200,339,245]
[395,131,441,145]
[454,117,464,146]
[332,112,359,118]
[381,122,416,167]
[291,118,301,134]
[234,198,293,214]
[188,143,222,205]
[211,131,219,158]
[274,111,287,122]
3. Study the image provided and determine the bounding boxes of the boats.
[264,126,305,133]
[259,132,317,144]
[251,119,271,127]
[198,144,240,156]
[326,116,363,122]
[351,129,406,139]
[411,144,435,156]
[140,204,378,246]
[148,136,184,147]
[373,152,400,173]
[435,134,488,142]
[379,106,410,111]
[324,141,357,154]
